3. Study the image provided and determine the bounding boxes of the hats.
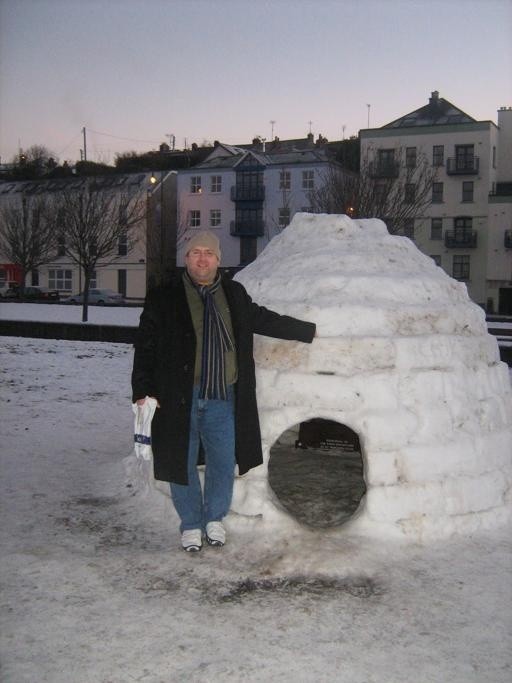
[184,228,222,262]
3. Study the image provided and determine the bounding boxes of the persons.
[132,230,318,553]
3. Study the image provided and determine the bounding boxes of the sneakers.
[181,528,204,551]
[205,520,227,547]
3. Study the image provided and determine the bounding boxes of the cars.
[65,288,122,306]
[0,281,48,297]
